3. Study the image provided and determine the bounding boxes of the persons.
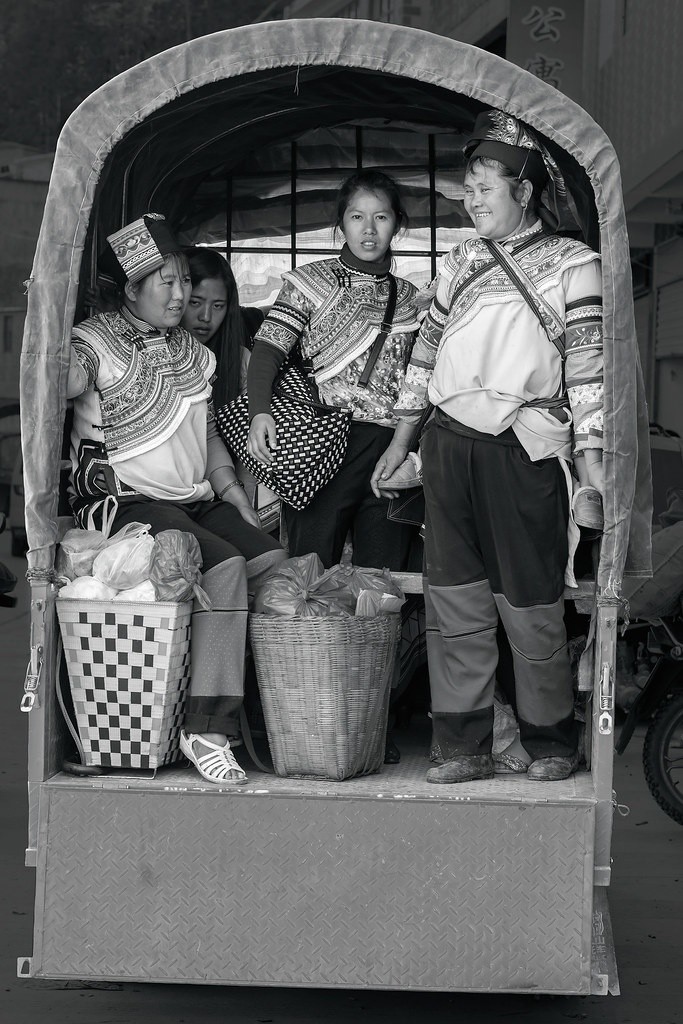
[63,130,605,785]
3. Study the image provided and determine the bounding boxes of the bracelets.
[218,479,245,501]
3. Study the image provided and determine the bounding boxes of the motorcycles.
[615,620,683,825]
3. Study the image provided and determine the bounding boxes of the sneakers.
[571,483,603,529]
[378,452,423,489]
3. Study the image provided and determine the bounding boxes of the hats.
[462,110,567,233]
[106,213,184,284]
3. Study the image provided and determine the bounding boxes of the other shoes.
[429,744,444,763]
[528,750,578,781]
[494,755,530,774]
[427,753,495,783]
[384,737,400,763]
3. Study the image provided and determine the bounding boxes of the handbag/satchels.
[215,350,354,512]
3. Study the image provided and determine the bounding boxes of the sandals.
[179,729,248,784]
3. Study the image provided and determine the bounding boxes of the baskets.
[55,596,193,769]
[251,612,400,780]
